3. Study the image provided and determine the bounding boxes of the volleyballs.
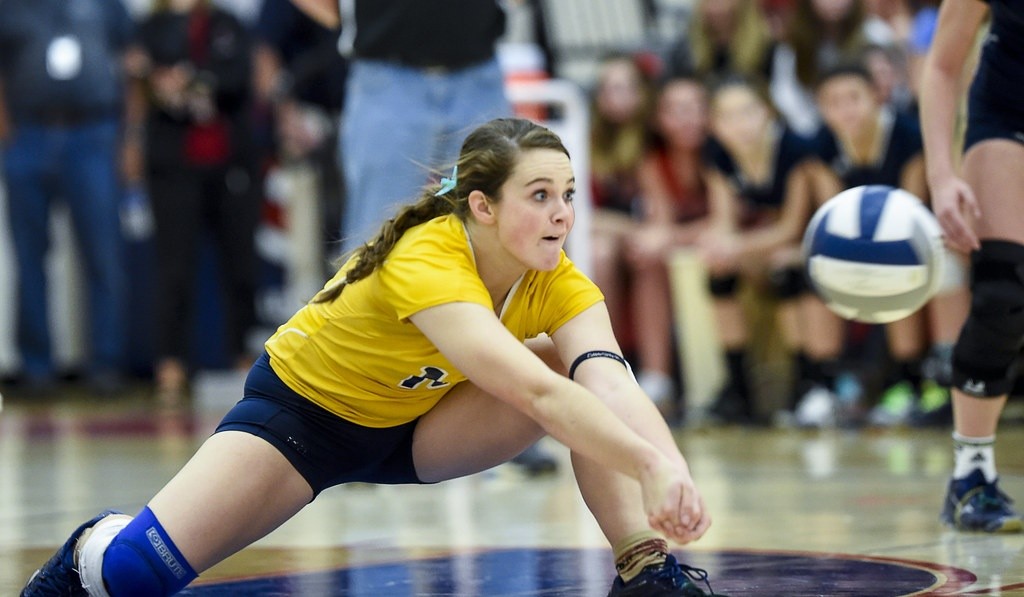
[802,184,946,324]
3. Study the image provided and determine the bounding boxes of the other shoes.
[88,368,139,401]
[775,387,809,429]
[867,381,913,426]
[634,371,684,422]
[797,372,862,430]
[194,368,249,393]
[912,375,955,429]
[699,379,759,427]
[23,373,78,400]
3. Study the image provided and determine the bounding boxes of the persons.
[19,118,727,597]
[0,0,1024,535]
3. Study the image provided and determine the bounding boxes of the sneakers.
[606,553,730,597]
[937,468,1024,533]
[19,510,121,597]
